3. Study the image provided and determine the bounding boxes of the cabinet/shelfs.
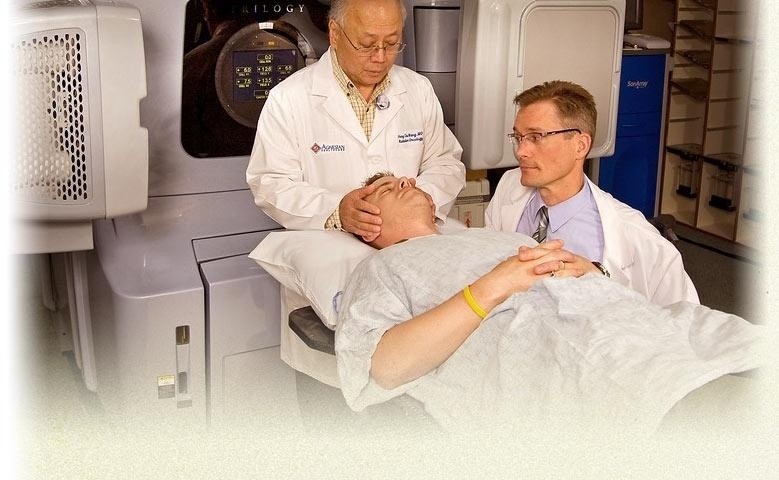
[596,53,667,219]
[659,0,762,253]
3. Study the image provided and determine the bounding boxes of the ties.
[531,206,548,244]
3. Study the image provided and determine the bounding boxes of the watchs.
[592,261,610,277]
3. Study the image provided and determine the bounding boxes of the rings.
[560,261,565,270]
[549,271,555,278]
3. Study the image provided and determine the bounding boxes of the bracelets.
[463,286,487,317]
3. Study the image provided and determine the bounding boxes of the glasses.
[508,128,581,144]
[336,22,406,57]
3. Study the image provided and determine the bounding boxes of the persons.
[484,79,701,307]
[183,0,243,94]
[335,171,768,440]
[245,2,467,439]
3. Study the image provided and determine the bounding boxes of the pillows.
[249,211,465,333]
